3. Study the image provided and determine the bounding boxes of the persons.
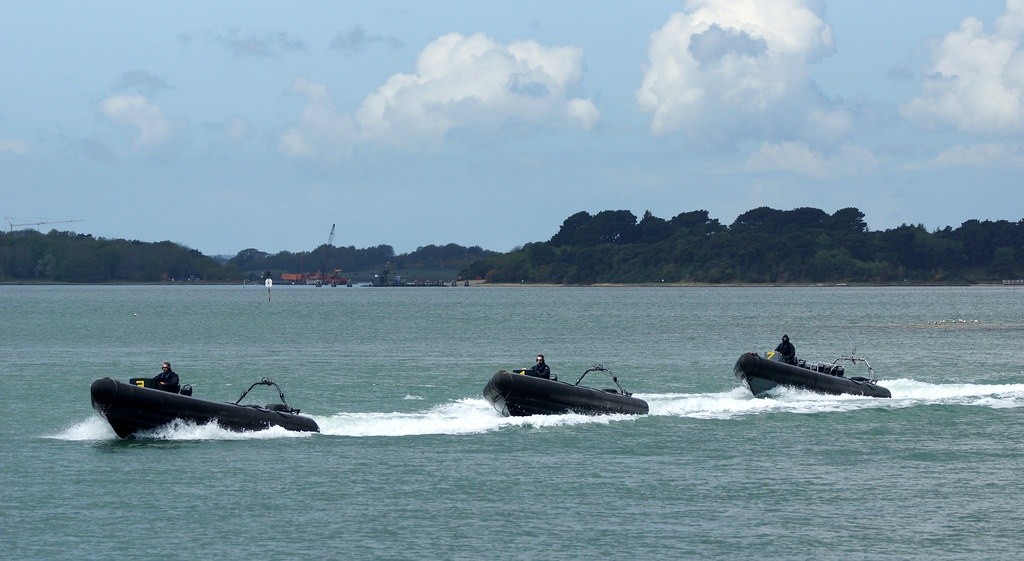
[154,362,179,392]
[774,334,795,365]
[522,355,550,379]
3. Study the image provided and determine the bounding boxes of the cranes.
[4,217,86,233]
[325,224,336,248]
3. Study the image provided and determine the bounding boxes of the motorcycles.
[483,364,652,417]
[89,376,323,439]
[731,350,894,402]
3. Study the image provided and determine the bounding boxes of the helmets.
[181,385,192,395]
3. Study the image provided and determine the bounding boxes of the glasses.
[536,359,542,361]
[162,367,169,369]
